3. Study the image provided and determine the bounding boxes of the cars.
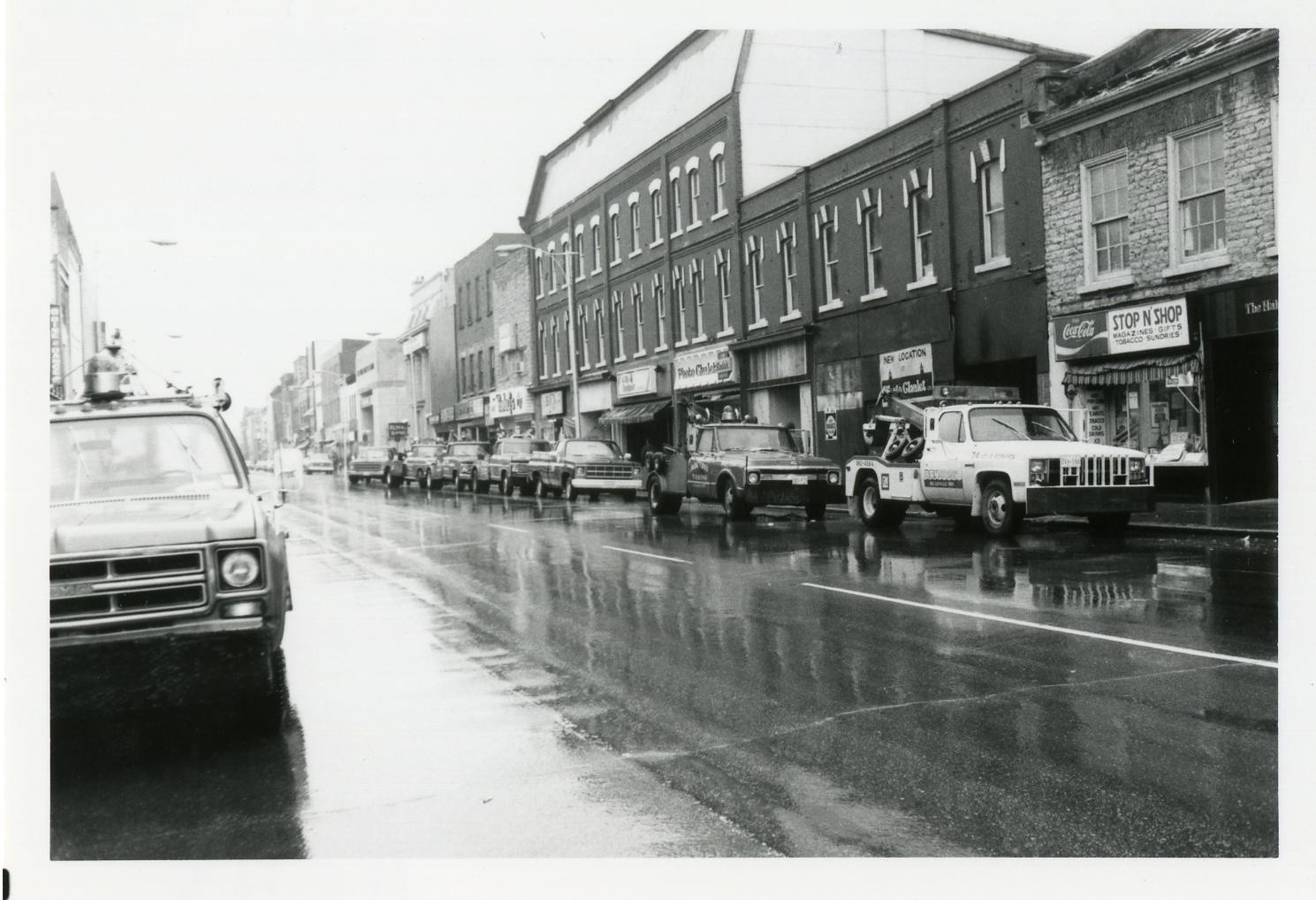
[244,458,274,471]
[303,452,335,475]
[50,356,294,686]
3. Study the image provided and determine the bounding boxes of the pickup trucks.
[641,422,844,521]
[844,385,1157,539]
[526,438,641,504]
[348,435,555,496]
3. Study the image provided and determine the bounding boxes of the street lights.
[315,369,348,479]
[368,330,419,441]
[495,243,580,440]
